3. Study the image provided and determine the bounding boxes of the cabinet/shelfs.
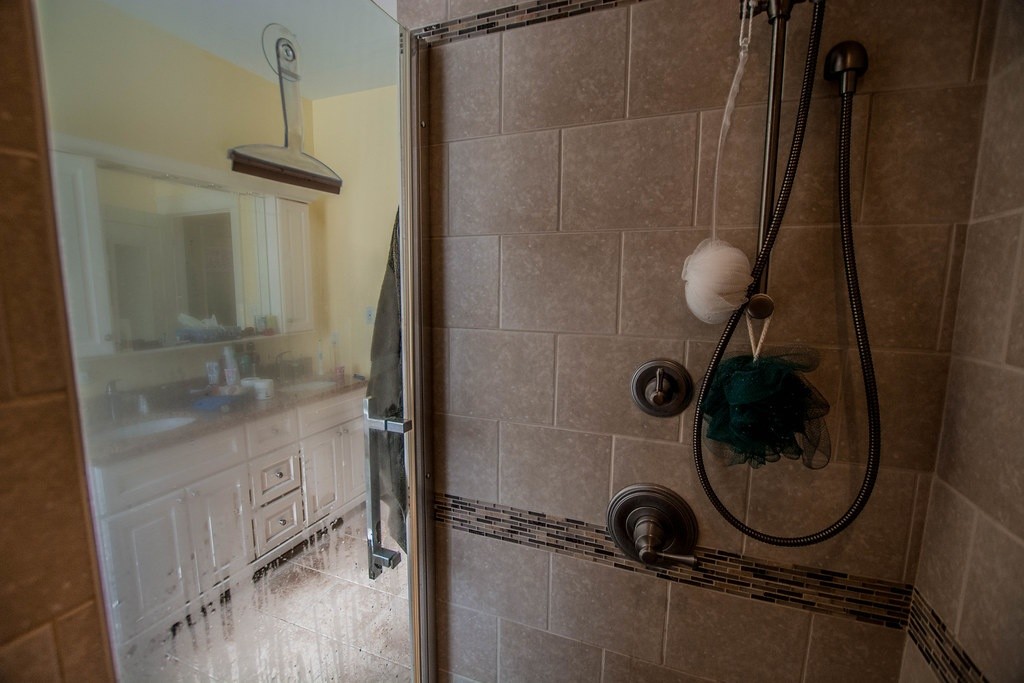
[245,409,304,566]
[87,426,257,657]
[297,386,367,531]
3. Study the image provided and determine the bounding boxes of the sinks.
[277,381,336,392]
[93,412,197,442]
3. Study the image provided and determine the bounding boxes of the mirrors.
[94,158,272,353]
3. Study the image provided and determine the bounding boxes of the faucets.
[275,351,292,382]
[106,377,123,423]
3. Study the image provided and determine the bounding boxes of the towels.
[367,203,407,552]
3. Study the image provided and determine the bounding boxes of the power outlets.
[365,305,374,324]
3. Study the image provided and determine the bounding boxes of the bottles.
[246,342,260,382]
[223,346,241,386]
[317,339,325,376]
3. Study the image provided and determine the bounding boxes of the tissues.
[177,312,240,344]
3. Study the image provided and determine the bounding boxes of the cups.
[223,368,241,385]
[335,366,345,390]
[240,377,261,398]
[254,378,274,400]
[206,362,221,385]
